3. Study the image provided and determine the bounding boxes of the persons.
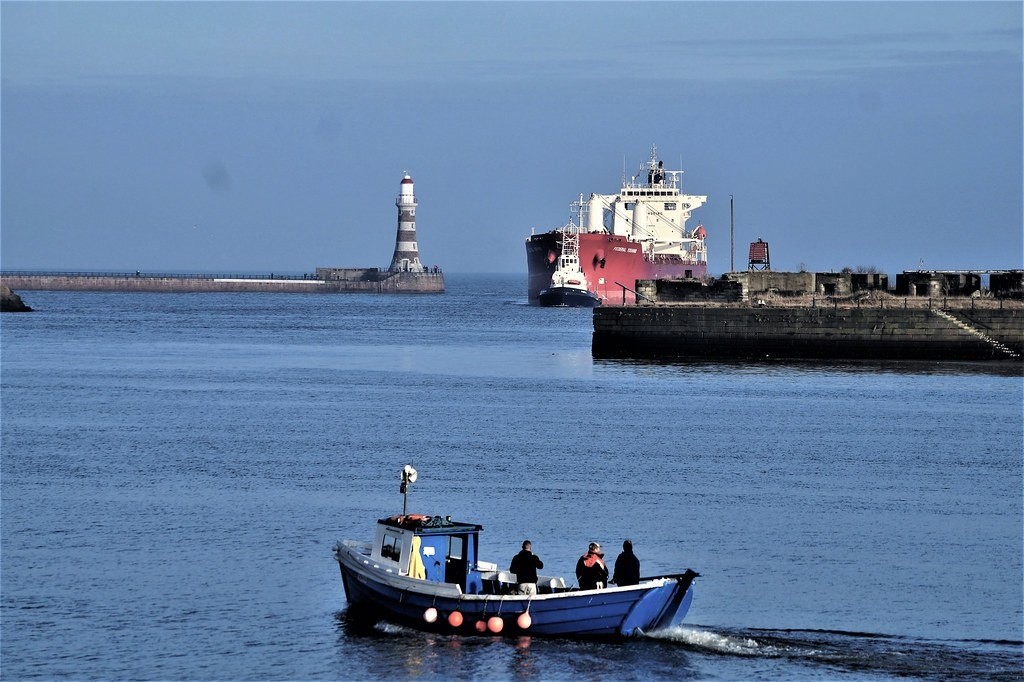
[608,540,640,587]
[575,542,608,590]
[509,540,544,595]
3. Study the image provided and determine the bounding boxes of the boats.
[333,463,703,640]
[536,216,603,308]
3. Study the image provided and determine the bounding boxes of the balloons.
[487,617,504,632]
[449,611,463,627]
[476,620,486,631]
[424,608,438,623]
[518,611,532,629]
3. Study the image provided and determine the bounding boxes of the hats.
[589,542,603,550]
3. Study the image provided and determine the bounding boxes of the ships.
[527,141,709,308]
